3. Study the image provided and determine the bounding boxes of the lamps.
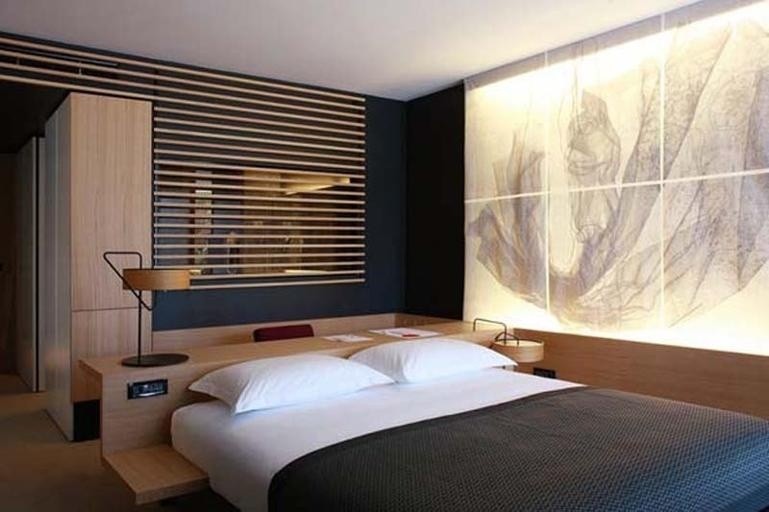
[103,251,191,368]
[472,316,545,363]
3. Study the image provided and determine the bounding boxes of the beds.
[78,320,769,512]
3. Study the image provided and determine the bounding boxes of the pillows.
[188,352,396,415]
[349,336,518,383]
